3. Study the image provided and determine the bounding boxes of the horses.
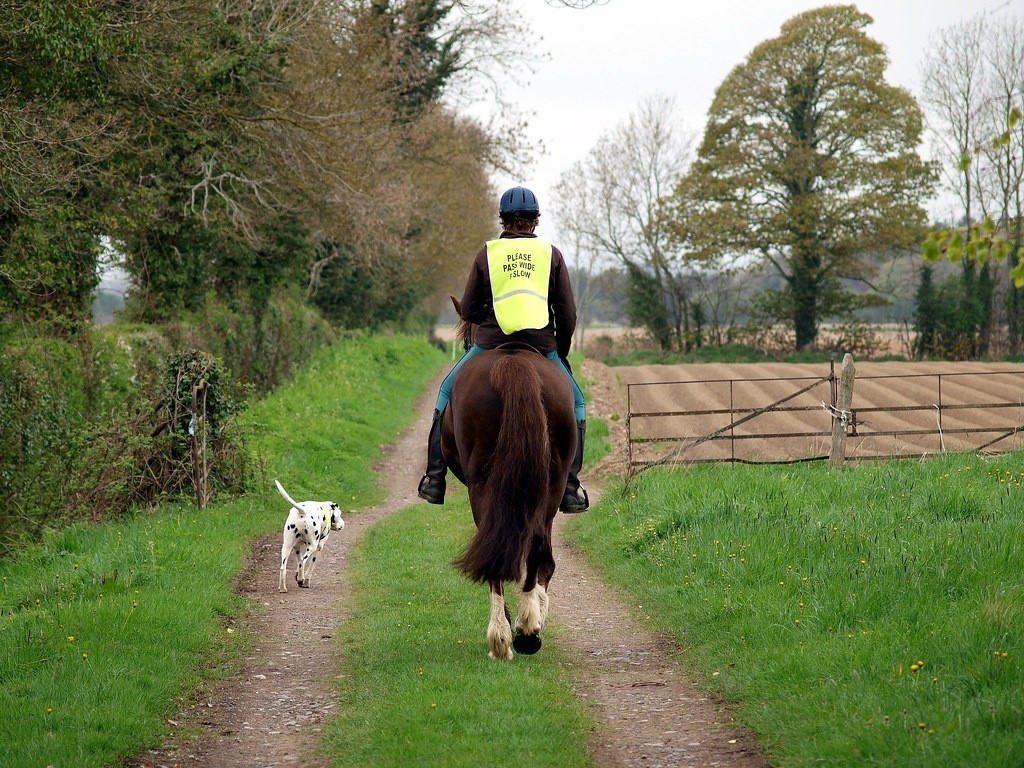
[440,292,582,662]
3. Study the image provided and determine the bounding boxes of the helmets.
[498,184,541,215]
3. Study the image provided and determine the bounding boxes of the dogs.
[274,479,345,594]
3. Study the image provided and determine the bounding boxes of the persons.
[417,186,590,514]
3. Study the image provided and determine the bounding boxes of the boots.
[417,410,448,501]
[561,421,587,510]
